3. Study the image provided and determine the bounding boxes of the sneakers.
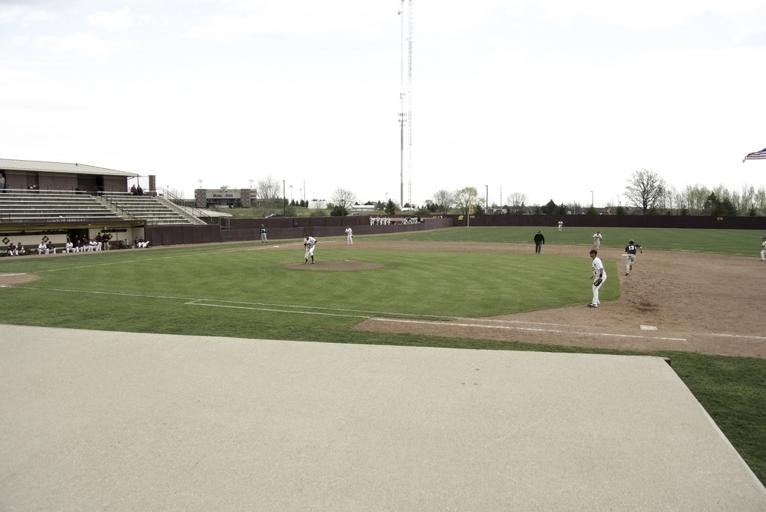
[588,302,600,308]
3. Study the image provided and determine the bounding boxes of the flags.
[744,148,766,163]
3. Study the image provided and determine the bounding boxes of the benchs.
[0,189,193,254]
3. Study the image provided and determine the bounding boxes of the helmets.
[629,239,635,244]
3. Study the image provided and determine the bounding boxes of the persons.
[135,237,143,248]
[303,232,317,264]
[259,223,269,242]
[558,219,564,231]
[140,237,150,248]
[15,242,26,256]
[587,249,608,308]
[136,184,144,195]
[8,243,19,256]
[624,239,643,276]
[344,223,353,244]
[534,230,545,254]
[760,236,766,261]
[130,184,138,195]
[37,230,110,256]
[0,173,5,193]
[593,231,602,249]
[368,216,421,226]
